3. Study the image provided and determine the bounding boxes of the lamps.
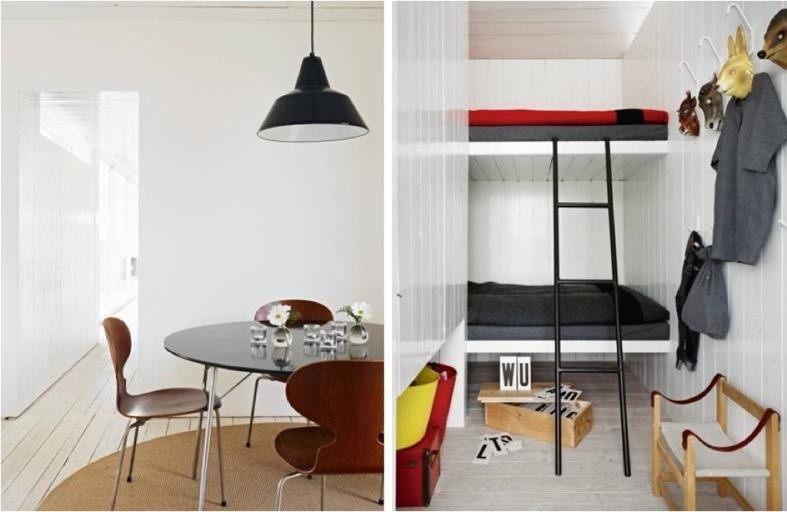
[253,1,373,144]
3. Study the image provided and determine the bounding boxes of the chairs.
[271,358,387,511]
[101,315,229,511]
[245,299,336,451]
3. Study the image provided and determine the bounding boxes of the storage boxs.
[396,426,441,507]
[477,378,595,449]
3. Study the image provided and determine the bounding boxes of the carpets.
[32,421,387,511]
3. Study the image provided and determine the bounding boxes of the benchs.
[650,373,782,511]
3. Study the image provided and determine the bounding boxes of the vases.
[271,326,292,348]
[348,323,369,345]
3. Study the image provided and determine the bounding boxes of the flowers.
[334,301,374,324]
[265,303,292,327]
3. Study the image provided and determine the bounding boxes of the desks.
[162,317,385,482]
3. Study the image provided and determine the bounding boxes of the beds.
[464,277,674,355]
[460,103,671,185]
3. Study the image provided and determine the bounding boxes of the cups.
[317,329,339,348]
[337,339,346,354]
[249,345,267,359]
[303,342,319,357]
[302,323,319,342]
[329,321,347,339]
[320,348,337,360]
[250,324,267,343]
